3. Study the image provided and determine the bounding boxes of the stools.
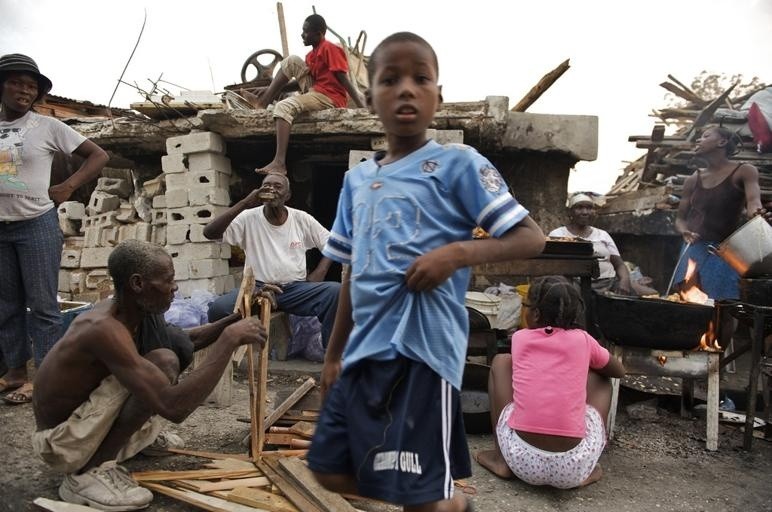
[460,330,498,414]
[714,300,772,451]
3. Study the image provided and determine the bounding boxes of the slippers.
[0,378,29,393]
[2,383,34,405]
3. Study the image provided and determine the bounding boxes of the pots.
[707,213,772,279]
[592,286,717,352]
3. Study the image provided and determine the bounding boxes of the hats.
[0,54,52,102]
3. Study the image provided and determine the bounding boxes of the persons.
[31,238,283,512]
[0,54,110,404]
[240,13,364,174]
[203,172,343,355]
[305,31,543,511]
[471,272,627,489]
[674,125,772,382]
[547,191,660,357]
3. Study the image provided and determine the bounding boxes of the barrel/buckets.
[760,357,772,426]
[464,290,502,328]
[515,283,529,330]
[25,299,92,369]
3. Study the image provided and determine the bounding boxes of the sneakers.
[140,431,185,456]
[58,458,154,512]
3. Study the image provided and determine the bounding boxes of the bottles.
[258,192,275,200]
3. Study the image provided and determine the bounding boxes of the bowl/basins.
[226,89,255,110]
[458,387,494,436]
